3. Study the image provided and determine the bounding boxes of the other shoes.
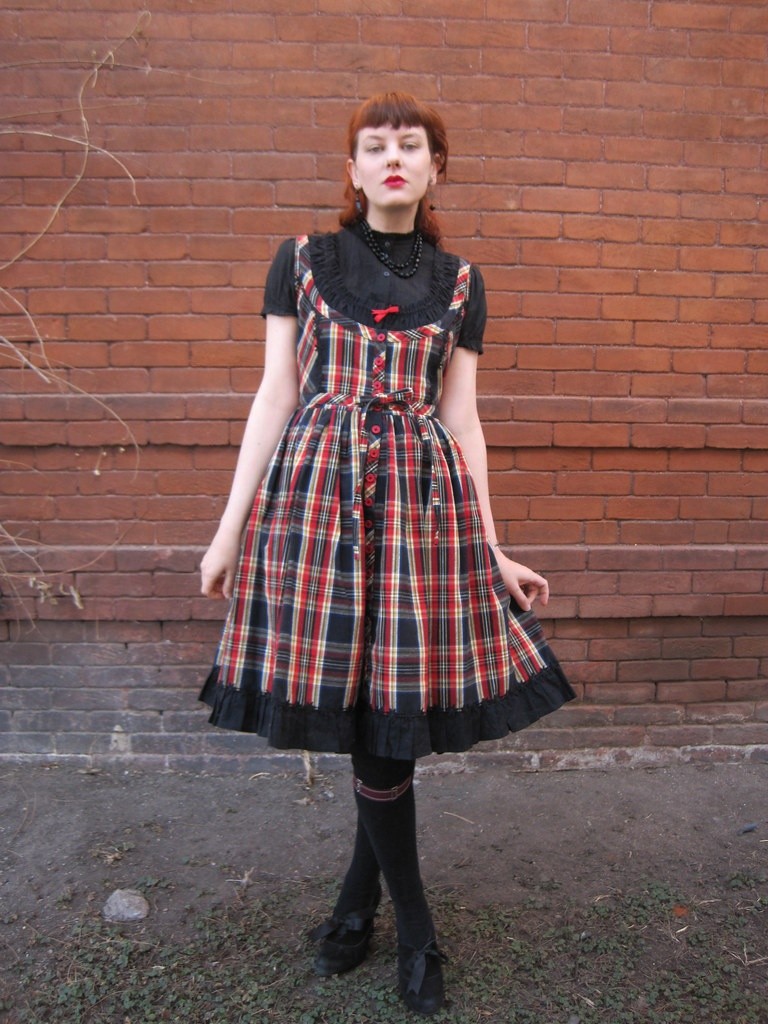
[396,927,448,1015]
[317,883,384,976]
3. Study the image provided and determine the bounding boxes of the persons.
[197,90,579,1017]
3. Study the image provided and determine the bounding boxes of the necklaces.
[359,219,423,279]
